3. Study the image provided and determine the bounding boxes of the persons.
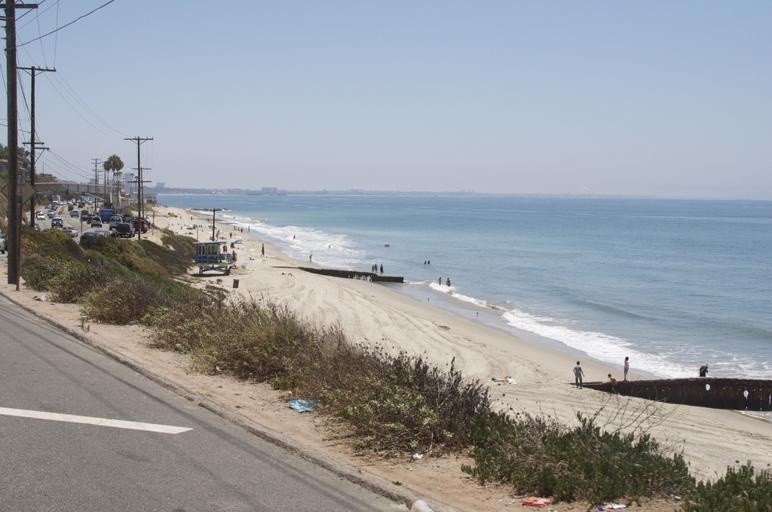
[380,264,384,274]
[261,243,265,256]
[700,365,705,377]
[446,277,450,286]
[216,230,243,261]
[439,277,442,285]
[371,264,375,272]
[608,374,617,394]
[573,361,584,389]
[624,357,629,382]
[310,254,312,262]
[375,263,378,273]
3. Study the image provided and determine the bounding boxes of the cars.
[36,195,149,242]
